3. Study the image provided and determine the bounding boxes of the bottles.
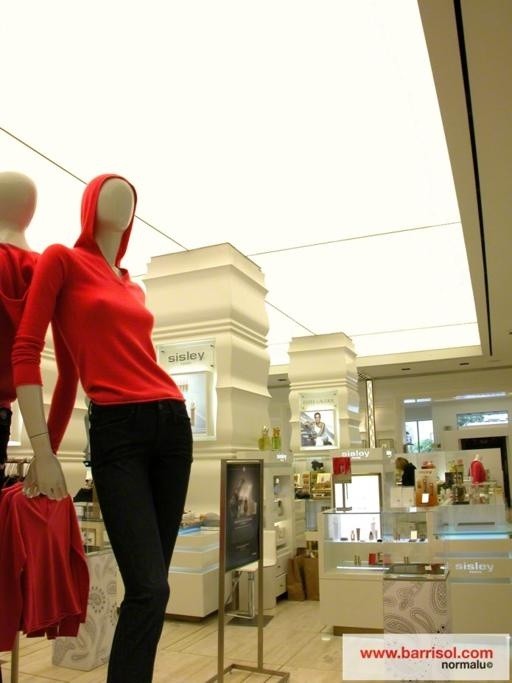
[272,425,283,450]
[378,552,384,565]
[370,522,377,540]
[354,555,357,567]
[358,555,362,566]
[257,424,272,451]
[369,531,374,540]
[356,528,360,541]
[274,477,281,500]
[351,530,355,541]
[438,470,503,505]
[414,462,439,506]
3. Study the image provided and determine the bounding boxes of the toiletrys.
[340,526,409,567]
[258,425,271,450]
[271,425,281,450]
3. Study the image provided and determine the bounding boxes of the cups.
[368,553,377,565]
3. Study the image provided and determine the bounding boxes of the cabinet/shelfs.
[316,506,438,636]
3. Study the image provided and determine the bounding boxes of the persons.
[305,411,326,445]
[0,169,81,487]
[394,455,417,486]
[468,452,487,484]
[452,472,462,484]
[15,171,195,680]
